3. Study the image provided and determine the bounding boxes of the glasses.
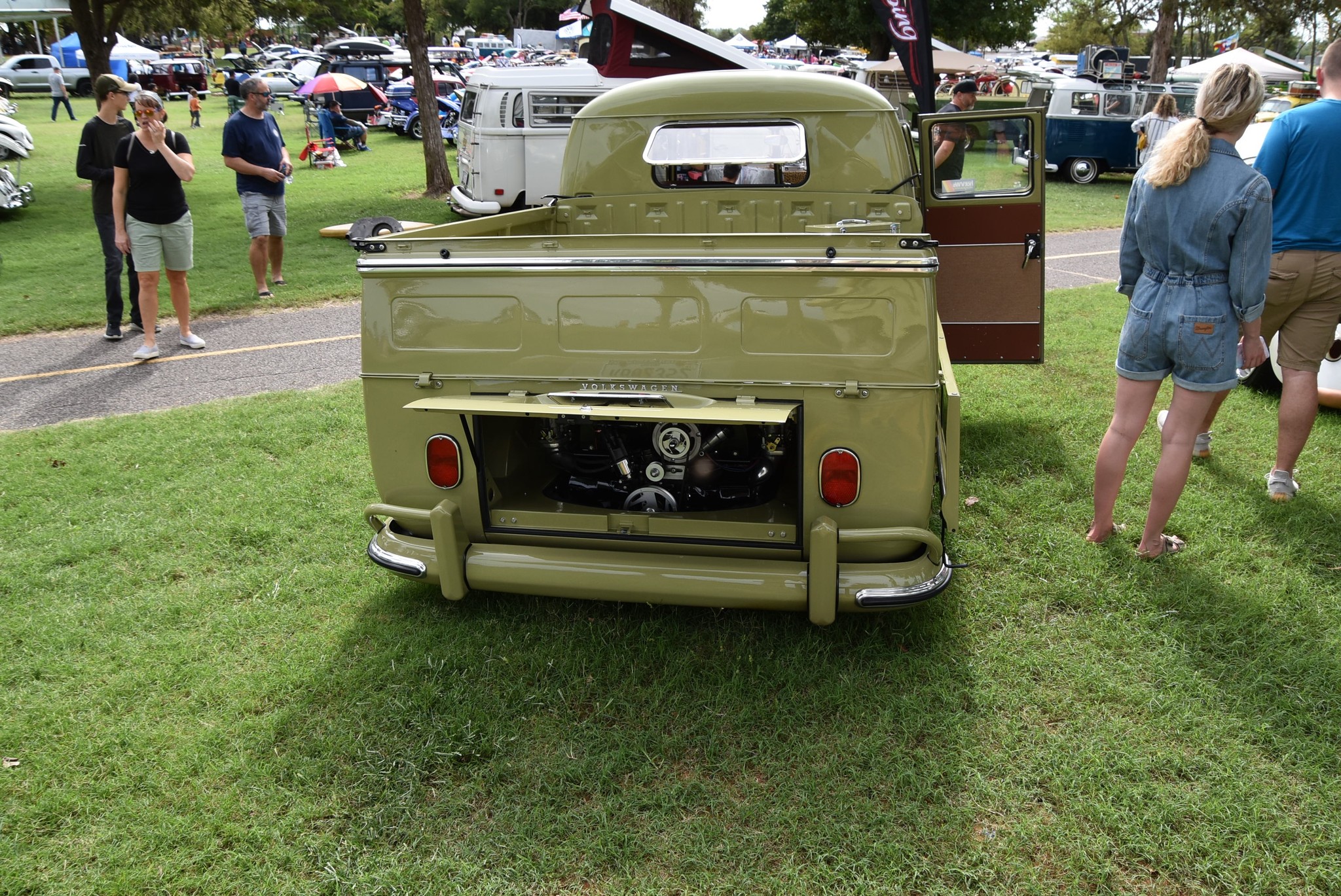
[132,108,162,119]
[248,92,269,97]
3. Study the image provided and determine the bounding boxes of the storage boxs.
[314,161,334,168]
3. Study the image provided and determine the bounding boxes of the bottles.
[281,164,293,185]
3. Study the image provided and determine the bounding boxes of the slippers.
[271,280,288,287]
[1137,532,1187,562]
[259,291,275,299]
[1086,523,1126,544]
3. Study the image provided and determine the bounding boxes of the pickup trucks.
[349,68,1049,627]
[0,54,94,97]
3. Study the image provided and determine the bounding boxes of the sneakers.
[129,313,162,333]
[103,321,123,339]
[180,331,206,348]
[1156,409,1213,458]
[1264,468,1300,503]
[358,142,373,152]
[133,343,160,359]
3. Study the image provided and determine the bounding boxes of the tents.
[51,28,161,90]
[1170,46,1303,88]
[860,48,1008,120]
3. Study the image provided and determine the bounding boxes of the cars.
[0,77,14,99]
[0,164,35,210]
[0,95,19,116]
[0,115,35,161]
[445,62,649,218]
[126,25,594,141]
[758,0,1341,185]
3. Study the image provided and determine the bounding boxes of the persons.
[110,91,206,360]
[139,21,585,65]
[1131,93,1180,166]
[921,80,984,193]
[221,78,294,301]
[224,70,241,119]
[1104,93,1125,115]
[327,100,372,152]
[185,85,199,127]
[1084,60,1276,559]
[190,90,202,128]
[720,163,742,185]
[1154,37,1341,500]
[48,67,78,122]
[721,33,898,79]
[146,83,158,96]
[75,72,162,341]
[126,73,143,125]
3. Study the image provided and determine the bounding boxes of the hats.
[94,73,137,97]
[953,78,984,94]
[329,100,342,108]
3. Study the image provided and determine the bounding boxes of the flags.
[558,4,590,20]
[1212,30,1240,54]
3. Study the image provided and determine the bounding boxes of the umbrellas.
[296,71,367,101]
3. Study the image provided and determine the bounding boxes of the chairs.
[302,98,358,152]
[304,125,336,168]
